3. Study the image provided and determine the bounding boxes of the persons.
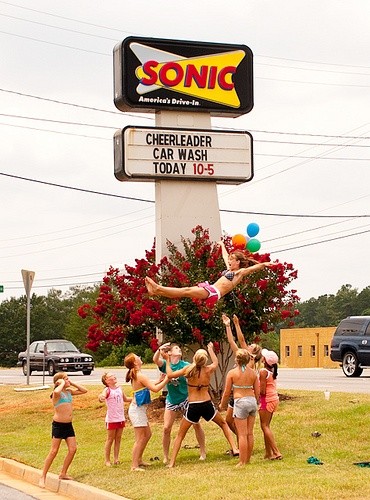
[219,349,260,465]
[124,353,172,471]
[98,371,133,465]
[222,314,262,455]
[145,242,277,304]
[39,372,88,488]
[153,342,206,464]
[258,348,283,460]
[162,341,239,468]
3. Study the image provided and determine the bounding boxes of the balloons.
[232,234,246,250]
[246,223,260,238]
[245,239,261,252]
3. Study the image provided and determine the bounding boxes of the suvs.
[329,317,370,378]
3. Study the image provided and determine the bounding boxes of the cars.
[19,339,94,376]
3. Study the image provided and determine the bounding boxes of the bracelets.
[225,324,230,327]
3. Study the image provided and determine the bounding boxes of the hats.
[261,349,278,367]
